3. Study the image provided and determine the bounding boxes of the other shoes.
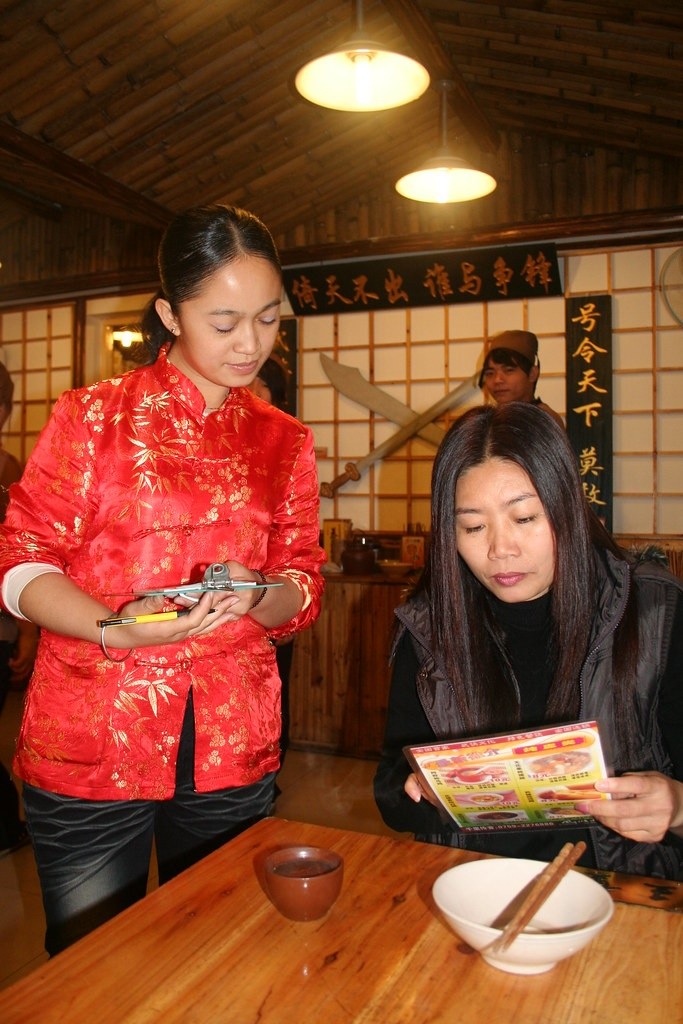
[0,820,31,857]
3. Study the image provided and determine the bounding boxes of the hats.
[483,329,538,365]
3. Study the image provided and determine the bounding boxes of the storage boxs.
[323,519,351,574]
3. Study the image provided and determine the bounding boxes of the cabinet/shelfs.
[288,583,416,761]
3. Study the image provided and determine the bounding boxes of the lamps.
[294,0,431,112]
[394,80,498,204]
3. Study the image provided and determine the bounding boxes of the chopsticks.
[493,841,585,952]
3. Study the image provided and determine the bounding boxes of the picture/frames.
[106,315,303,424]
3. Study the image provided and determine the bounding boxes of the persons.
[0,332,565,855]
[375,403,683,882]
[0,201,330,955]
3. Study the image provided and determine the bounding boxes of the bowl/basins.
[432,858,613,974]
[374,560,398,572]
[380,563,413,577]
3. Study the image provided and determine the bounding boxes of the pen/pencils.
[98,608,220,629]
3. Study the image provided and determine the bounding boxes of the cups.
[265,847,344,920]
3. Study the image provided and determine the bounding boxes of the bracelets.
[100,613,134,663]
[250,567,268,612]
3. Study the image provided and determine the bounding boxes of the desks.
[0,816,682,1024]
